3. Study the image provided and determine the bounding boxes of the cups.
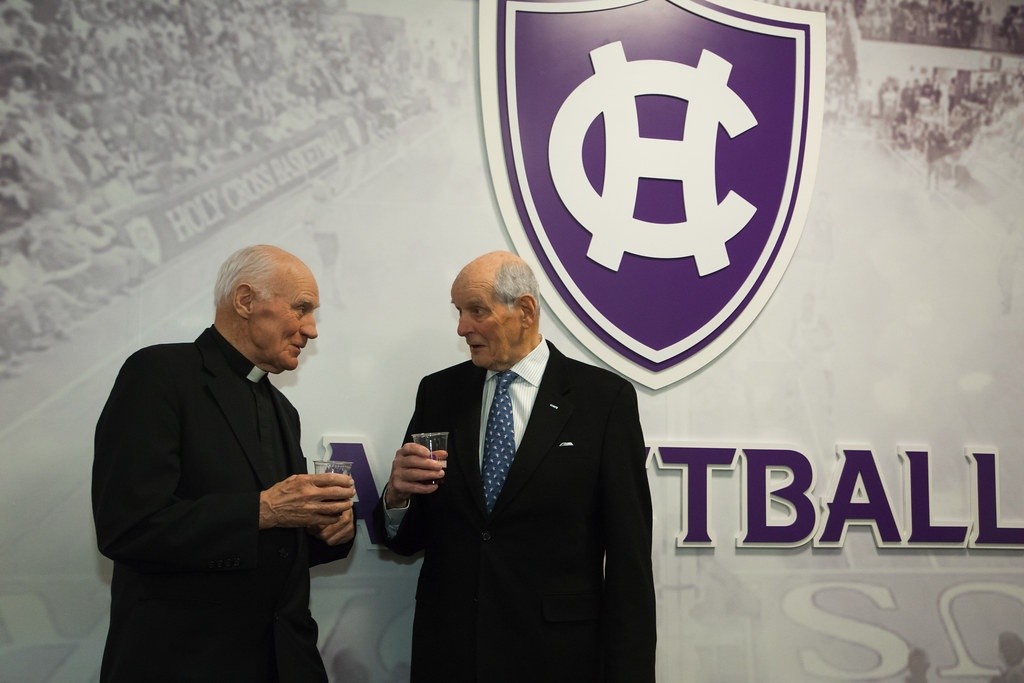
[411,430,449,485]
[311,459,354,516]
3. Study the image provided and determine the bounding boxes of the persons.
[370,250,656,683]
[90,244,354,682]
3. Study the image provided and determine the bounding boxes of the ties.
[481,370,519,514]
